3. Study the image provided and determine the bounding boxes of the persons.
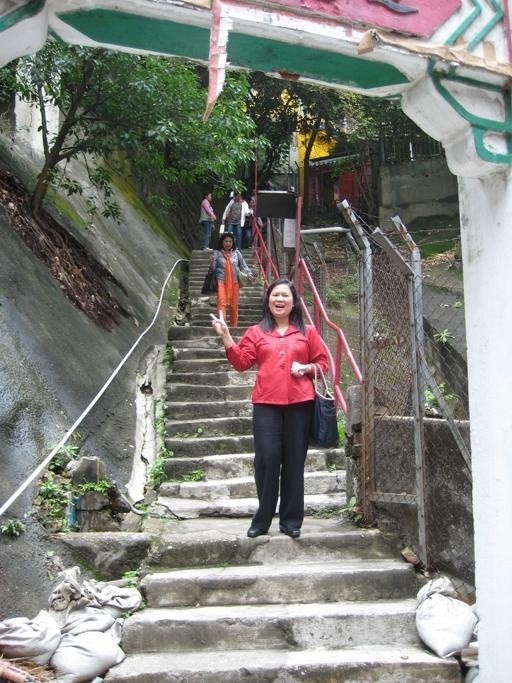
[209,279,330,538]
[198,192,217,251]
[222,187,256,250]
[207,232,254,329]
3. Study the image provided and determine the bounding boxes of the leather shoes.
[246,524,265,538]
[279,523,301,538]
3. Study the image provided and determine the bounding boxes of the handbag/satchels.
[200,265,220,297]
[219,222,226,234]
[211,220,216,232]
[305,359,341,451]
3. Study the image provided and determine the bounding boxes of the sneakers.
[203,247,214,251]
